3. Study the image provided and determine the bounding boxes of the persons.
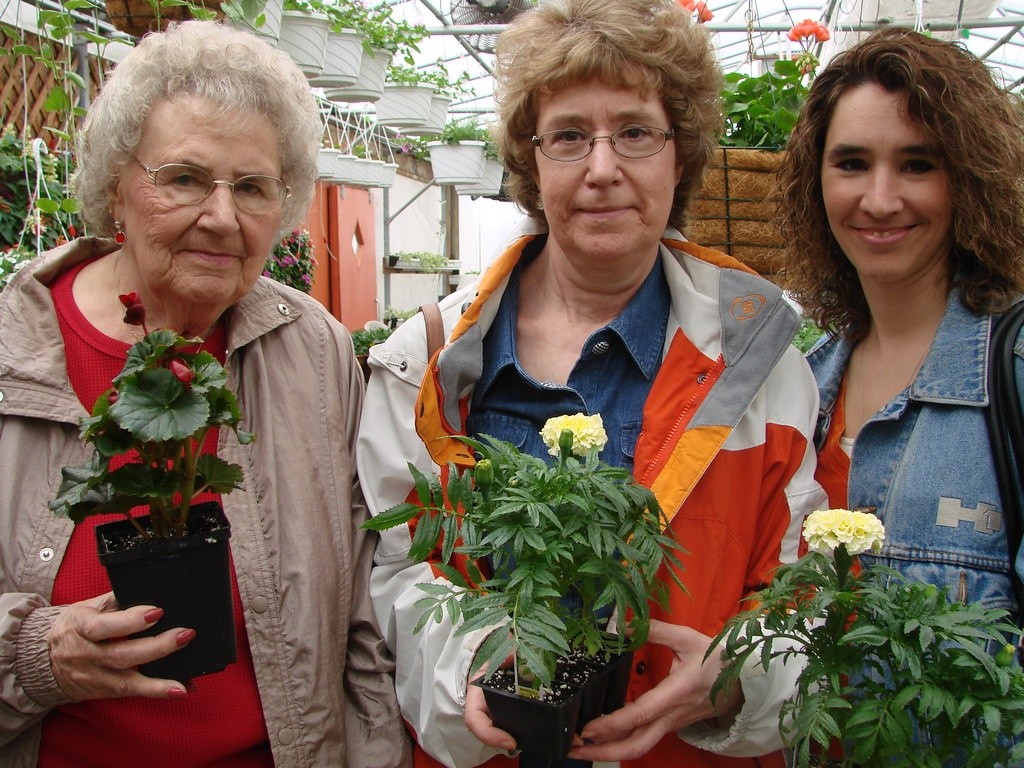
[0,18,413,768]
[357,0,838,768]
[777,27,1023,755]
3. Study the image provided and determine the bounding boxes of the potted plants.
[384,252,462,273]
[351,328,395,369]
[376,58,478,135]
[383,306,418,330]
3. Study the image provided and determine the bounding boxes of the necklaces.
[112,262,139,341]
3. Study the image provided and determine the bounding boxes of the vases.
[280,11,331,78]
[427,141,486,184]
[307,27,370,88]
[318,98,399,188]
[455,161,504,196]
[471,639,633,756]
[94,500,237,681]
[223,0,283,46]
[324,47,393,103]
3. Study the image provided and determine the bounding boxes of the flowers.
[282,0,430,65]
[359,412,691,695]
[48,293,257,540]
[674,0,831,152]
[320,136,340,150]
[391,117,500,163]
[352,145,373,159]
[700,509,1024,768]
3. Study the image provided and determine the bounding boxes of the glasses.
[124,150,293,216]
[531,126,676,163]
